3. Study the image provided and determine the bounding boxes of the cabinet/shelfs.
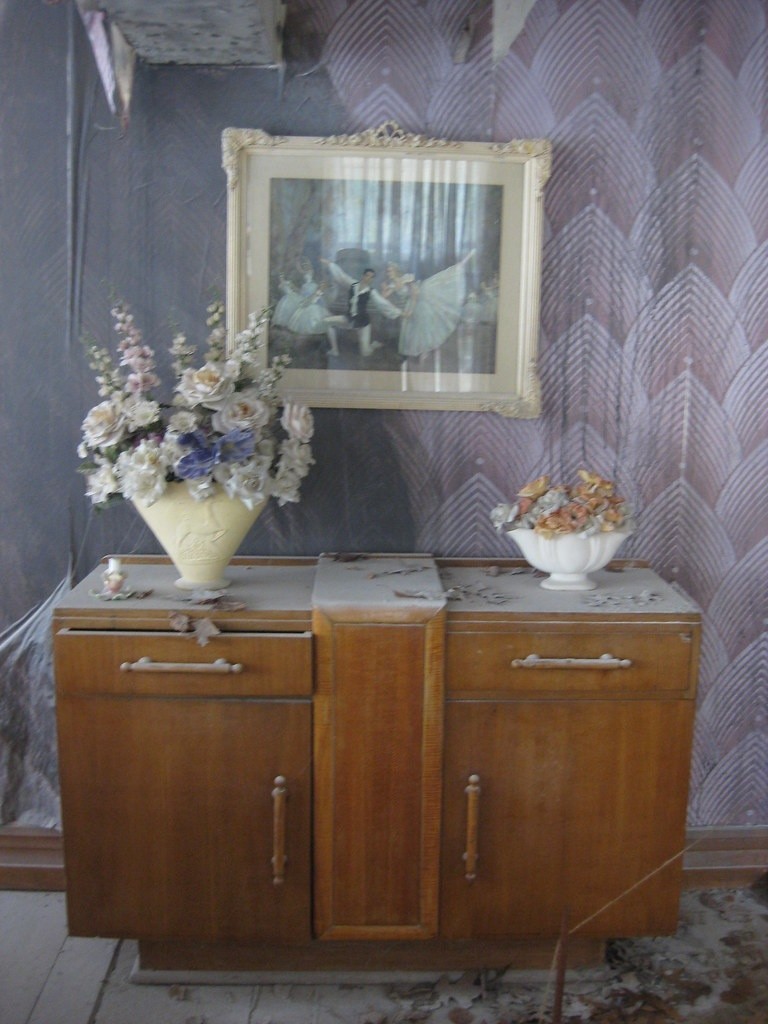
[49,553,704,981]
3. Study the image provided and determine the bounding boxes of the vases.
[505,527,633,589]
[131,477,270,593]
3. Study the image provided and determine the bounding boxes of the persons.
[382,246,481,365]
[315,258,411,358]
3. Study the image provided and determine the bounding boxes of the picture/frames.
[219,120,543,421]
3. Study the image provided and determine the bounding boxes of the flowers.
[490,469,639,540]
[76,279,319,508]
